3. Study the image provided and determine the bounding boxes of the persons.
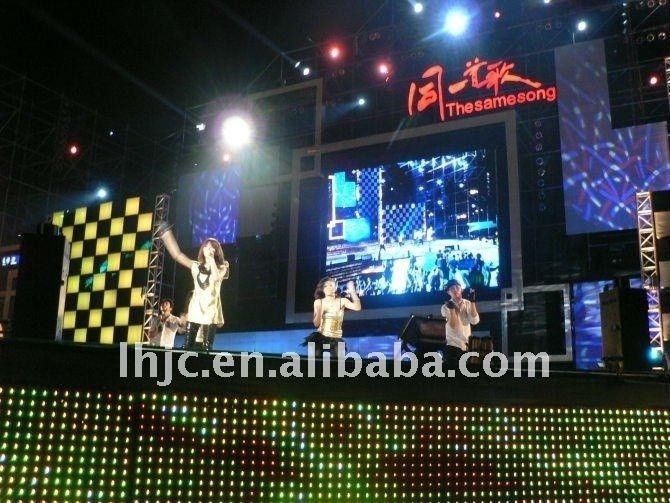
[145,295,187,345]
[300,274,363,358]
[325,219,500,295]
[155,220,231,353]
[178,288,209,350]
[440,278,480,367]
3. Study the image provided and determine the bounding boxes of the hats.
[445,278,461,286]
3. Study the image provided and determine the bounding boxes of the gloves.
[445,299,456,308]
[468,288,476,302]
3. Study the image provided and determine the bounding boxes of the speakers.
[598,287,650,371]
[601,1,670,129]
[11,235,73,341]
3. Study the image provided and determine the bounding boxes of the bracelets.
[352,298,363,302]
